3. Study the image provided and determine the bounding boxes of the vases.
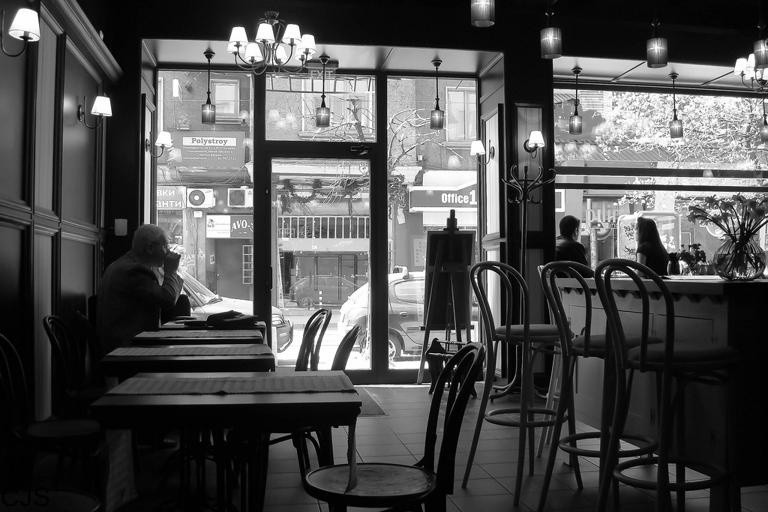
[713,229,767,280]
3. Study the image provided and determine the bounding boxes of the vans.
[176,270,293,354]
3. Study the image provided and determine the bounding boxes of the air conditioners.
[228,186,253,208]
[186,187,214,209]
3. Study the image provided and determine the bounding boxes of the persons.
[91,223,186,455]
[555,215,592,279]
[627,217,669,278]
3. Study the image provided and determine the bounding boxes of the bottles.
[707,265,714,274]
[700,265,706,274]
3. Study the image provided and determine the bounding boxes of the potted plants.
[666,252,680,276]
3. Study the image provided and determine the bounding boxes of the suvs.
[290,275,358,306]
[337,266,479,362]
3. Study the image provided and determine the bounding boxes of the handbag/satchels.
[208,311,256,329]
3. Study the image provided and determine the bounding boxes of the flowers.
[680,243,706,267]
[686,190,768,273]
